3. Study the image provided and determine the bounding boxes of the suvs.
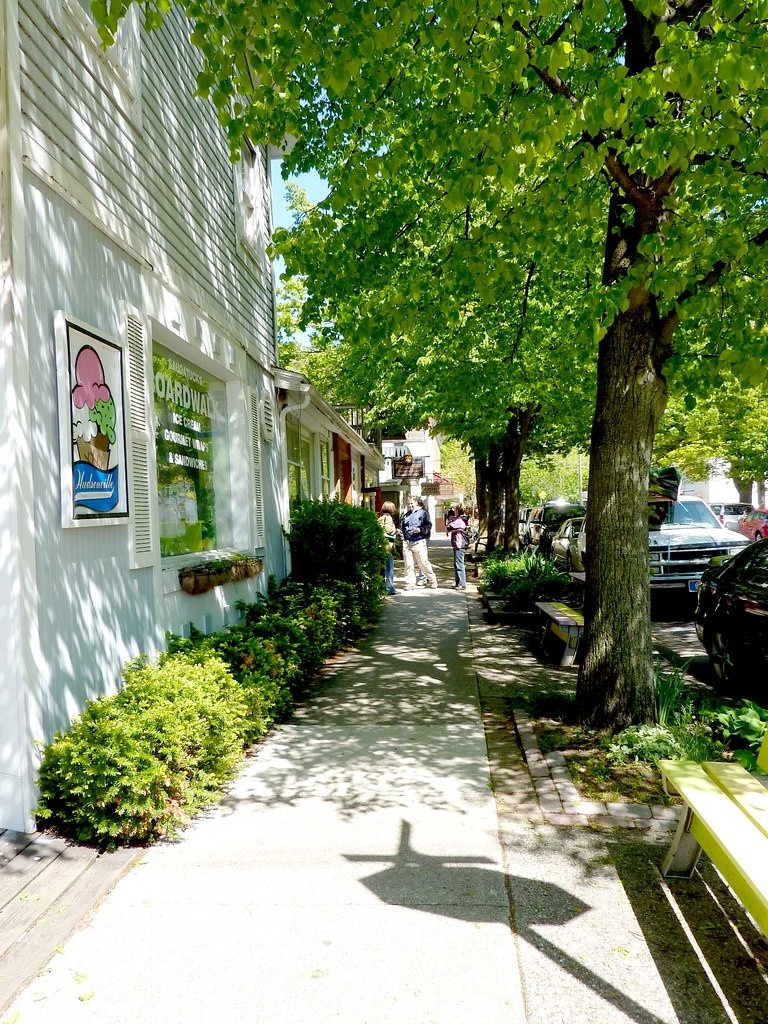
[579,495,753,620]
[517,501,584,560]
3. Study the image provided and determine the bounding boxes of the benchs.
[534,600,586,668]
[656,759,768,937]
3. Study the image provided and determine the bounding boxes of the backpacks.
[458,513,480,544]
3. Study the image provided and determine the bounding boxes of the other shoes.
[417,579,428,586]
[388,592,401,596]
[403,585,416,590]
[431,581,438,589]
[451,585,458,588]
[455,586,466,590]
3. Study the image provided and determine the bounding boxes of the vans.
[710,501,755,534]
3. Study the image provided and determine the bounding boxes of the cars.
[548,516,587,576]
[738,509,768,541]
[694,536,768,702]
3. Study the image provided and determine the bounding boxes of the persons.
[447,508,455,521]
[377,495,438,595]
[446,505,469,591]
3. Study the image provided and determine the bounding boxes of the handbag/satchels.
[381,515,396,543]
